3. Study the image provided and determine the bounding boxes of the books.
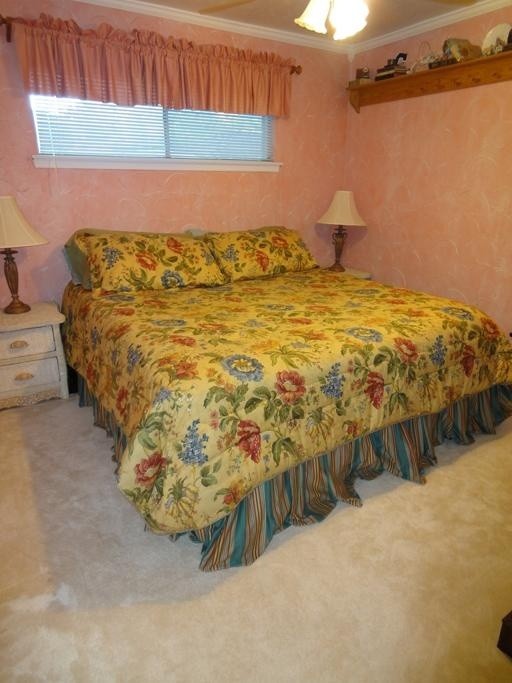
[377,64,405,72]
[376,68,408,76]
[426,56,457,69]
[374,72,411,81]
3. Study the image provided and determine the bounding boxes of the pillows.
[73,223,317,297]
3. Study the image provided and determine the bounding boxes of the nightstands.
[0,303,71,412]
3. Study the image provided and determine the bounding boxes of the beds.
[59,263,508,546]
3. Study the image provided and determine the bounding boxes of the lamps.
[292,0,331,38]
[0,192,49,315]
[317,190,366,271]
